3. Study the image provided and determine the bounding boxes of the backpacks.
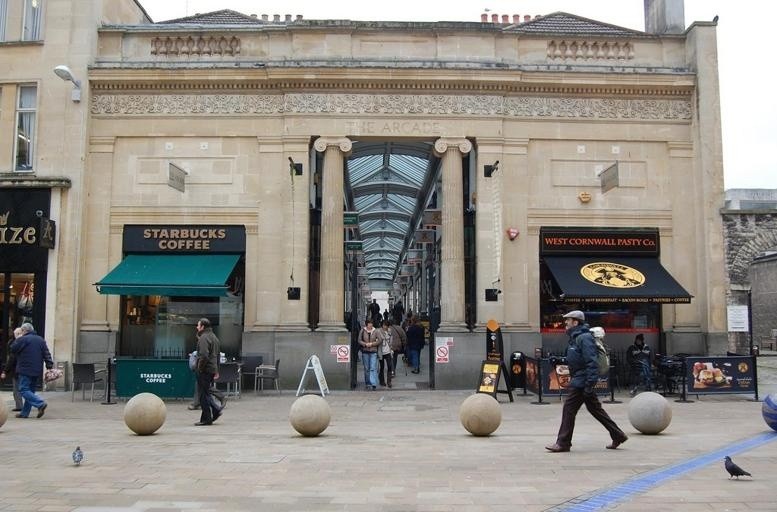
[569,327,610,377]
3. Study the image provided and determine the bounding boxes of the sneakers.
[37,402,48,418]
[220,396,228,409]
[188,404,201,410]
[15,413,29,418]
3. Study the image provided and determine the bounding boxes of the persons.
[589,325,611,376]
[357,297,425,391]
[10,279,34,330]
[540,309,630,452]
[193,316,222,424]
[11,322,54,418]
[188,325,229,410]
[626,333,655,395]
[8,327,24,412]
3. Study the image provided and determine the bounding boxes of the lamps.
[54,64,82,103]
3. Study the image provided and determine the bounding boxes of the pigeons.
[723,456,752,480]
[72,446,84,466]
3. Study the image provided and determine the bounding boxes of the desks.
[224,360,246,396]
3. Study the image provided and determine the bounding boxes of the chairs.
[544,345,703,400]
[240,354,264,392]
[105,361,126,403]
[70,359,107,404]
[253,358,284,398]
[216,361,244,402]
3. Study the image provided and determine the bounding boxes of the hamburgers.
[698,369,722,384]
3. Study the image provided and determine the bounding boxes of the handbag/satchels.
[43,368,64,384]
[390,348,395,359]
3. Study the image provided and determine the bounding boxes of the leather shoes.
[545,442,571,453]
[212,412,222,421]
[606,433,628,450]
[194,420,212,425]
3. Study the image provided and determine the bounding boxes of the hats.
[635,333,644,342]
[563,310,586,322]
